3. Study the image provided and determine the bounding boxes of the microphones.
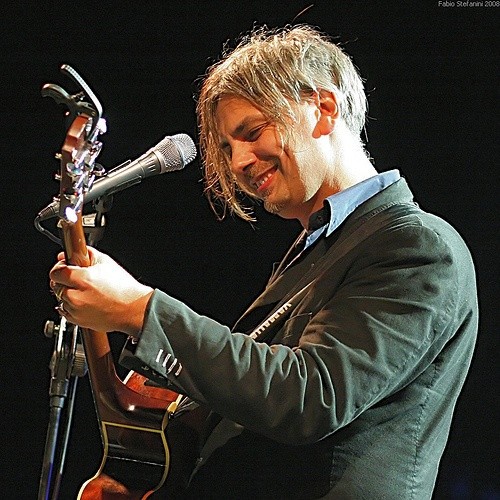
[38,134,198,221]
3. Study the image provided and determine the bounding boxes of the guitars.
[39,63,213,500]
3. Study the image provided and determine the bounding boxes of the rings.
[60,302,67,314]
[56,285,66,301]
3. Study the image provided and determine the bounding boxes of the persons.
[50,24,480,500]
